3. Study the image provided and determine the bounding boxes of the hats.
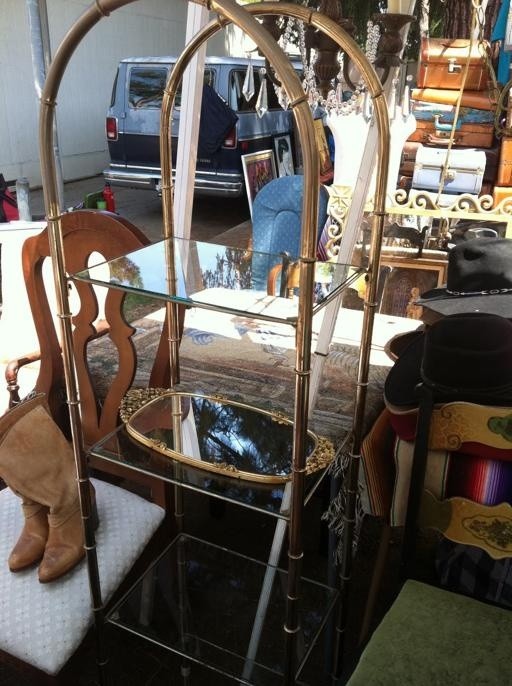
[410,236,512,321]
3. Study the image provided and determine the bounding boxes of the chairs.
[2,206,193,674]
[252,175,329,296]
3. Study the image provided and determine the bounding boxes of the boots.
[0,403,100,584]
[0,389,54,572]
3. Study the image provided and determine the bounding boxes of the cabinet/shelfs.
[35,0,392,682]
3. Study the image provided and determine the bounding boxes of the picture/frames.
[379,255,448,323]
[240,116,333,223]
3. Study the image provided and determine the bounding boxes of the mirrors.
[117,386,334,486]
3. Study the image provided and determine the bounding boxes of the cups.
[96,198,107,210]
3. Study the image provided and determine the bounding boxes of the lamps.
[255,0,416,113]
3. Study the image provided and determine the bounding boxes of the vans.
[102,54,335,197]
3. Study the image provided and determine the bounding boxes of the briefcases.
[410,87,504,111]
[408,99,499,124]
[405,119,496,147]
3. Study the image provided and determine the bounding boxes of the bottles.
[102,182,115,212]
